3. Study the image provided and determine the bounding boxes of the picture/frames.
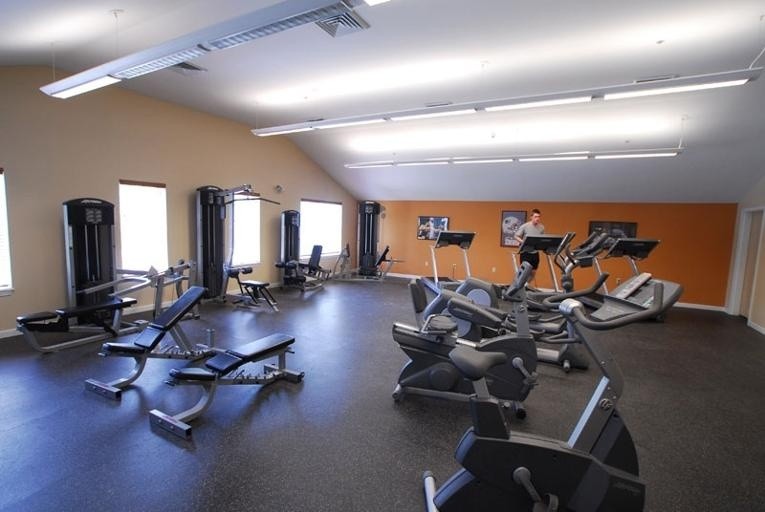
[501,211,527,248]
[416,216,449,240]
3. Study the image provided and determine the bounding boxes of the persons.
[514,208,545,287]
[419,219,441,239]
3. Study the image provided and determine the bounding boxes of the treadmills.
[492,236,567,312]
[574,239,660,310]
[421,232,475,295]
